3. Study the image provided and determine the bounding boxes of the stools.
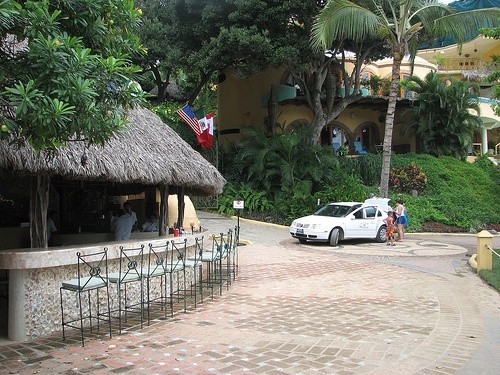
[60,224,240,346]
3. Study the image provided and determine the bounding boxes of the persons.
[46,201,137,246]
[382,211,397,246]
[393,199,409,242]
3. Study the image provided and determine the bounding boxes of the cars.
[289,201,390,247]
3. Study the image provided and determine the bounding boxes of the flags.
[176,104,203,136]
[195,112,213,148]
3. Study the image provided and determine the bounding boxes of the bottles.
[165,225,170,236]
